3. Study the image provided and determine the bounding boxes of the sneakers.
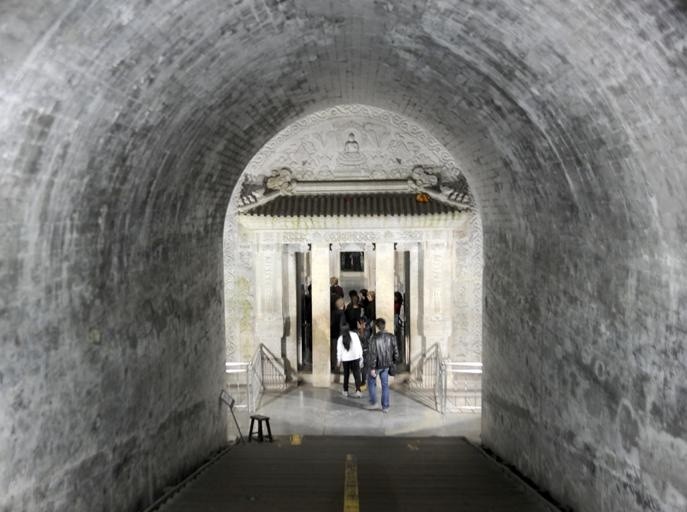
[362,403,390,413]
[360,384,367,392]
[351,390,362,398]
[342,390,349,397]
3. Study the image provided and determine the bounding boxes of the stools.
[248,414,275,442]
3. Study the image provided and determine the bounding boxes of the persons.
[365,318,402,412]
[336,322,364,399]
[301,276,405,374]
